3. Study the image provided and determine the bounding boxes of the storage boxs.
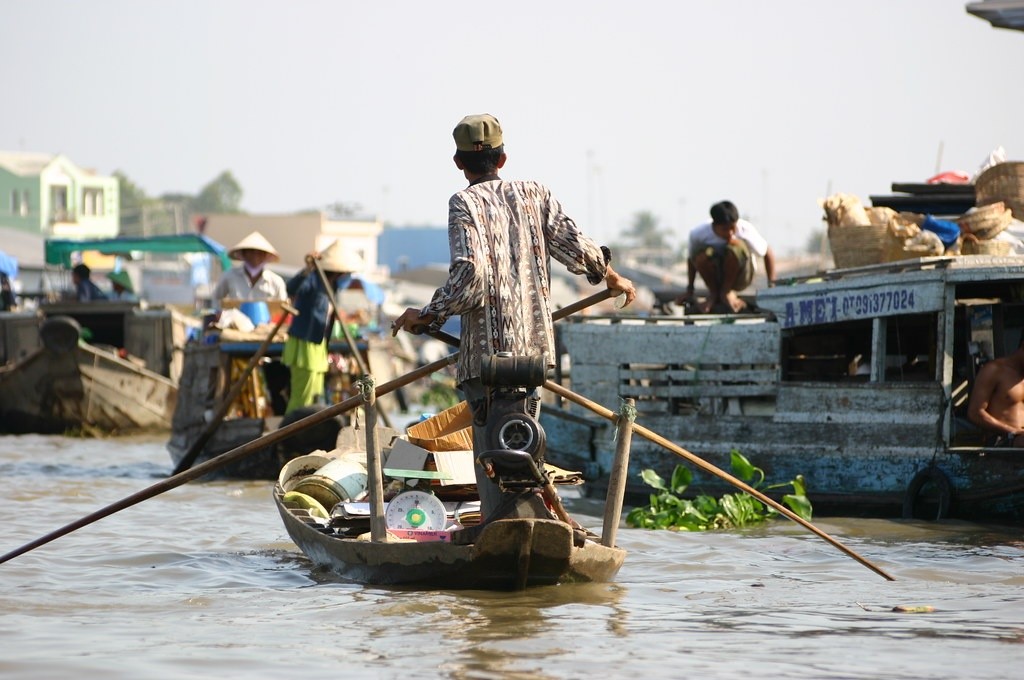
[406,400,475,486]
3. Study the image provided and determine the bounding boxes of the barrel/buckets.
[294,458,369,514]
[241,301,271,329]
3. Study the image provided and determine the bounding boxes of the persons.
[384,114,641,524]
[0,231,370,422]
[966,337,1024,457]
[681,201,779,318]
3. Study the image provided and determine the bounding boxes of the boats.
[533,255,1023,524]
[162,299,402,481]
[3,300,204,440]
[273,425,624,587]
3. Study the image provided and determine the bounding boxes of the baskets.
[827,161,1024,268]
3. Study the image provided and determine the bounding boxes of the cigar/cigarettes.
[389,319,400,328]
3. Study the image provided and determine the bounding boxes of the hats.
[453,114,503,151]
[313,238,359,272]
[226,231,279,264]
[106,270,134,293]
